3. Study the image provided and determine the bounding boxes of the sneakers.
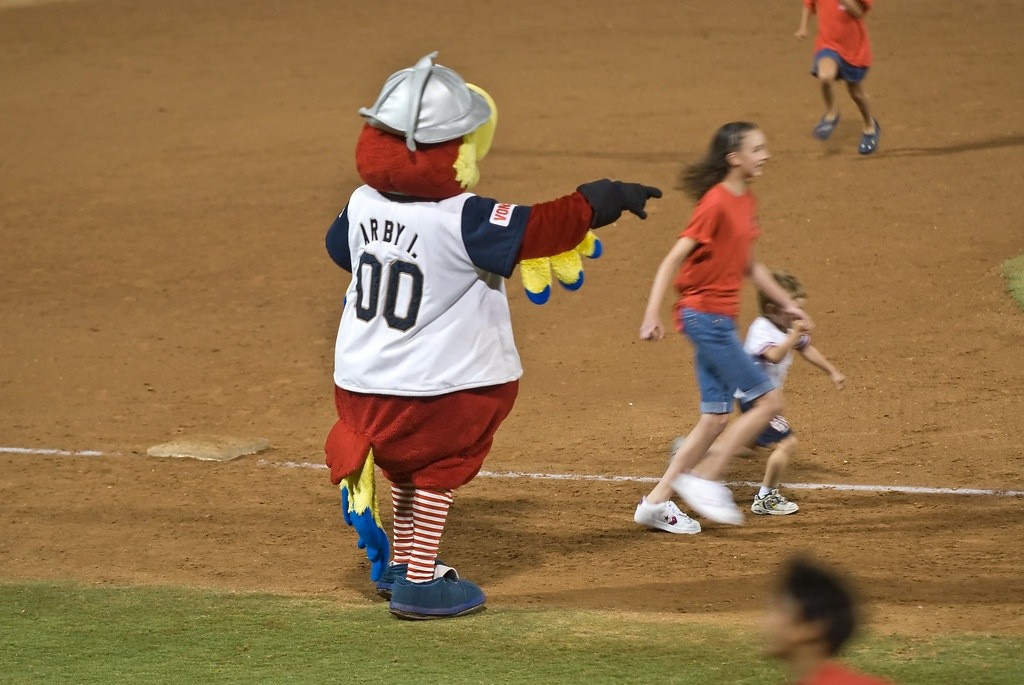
[634,495,701,535]
[671,473,745,526]
[751,488,799,515]
[670,438,683,459]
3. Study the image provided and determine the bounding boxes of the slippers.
[858,118,880,154]
[812,112,840,139]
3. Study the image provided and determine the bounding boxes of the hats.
[759,269,808,311]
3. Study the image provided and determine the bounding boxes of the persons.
[634,121,816,534]
[733,272,845,516]
[794,0,881,155]
[758,558,896,685]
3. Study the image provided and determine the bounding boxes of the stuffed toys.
[324,52,663,619]
